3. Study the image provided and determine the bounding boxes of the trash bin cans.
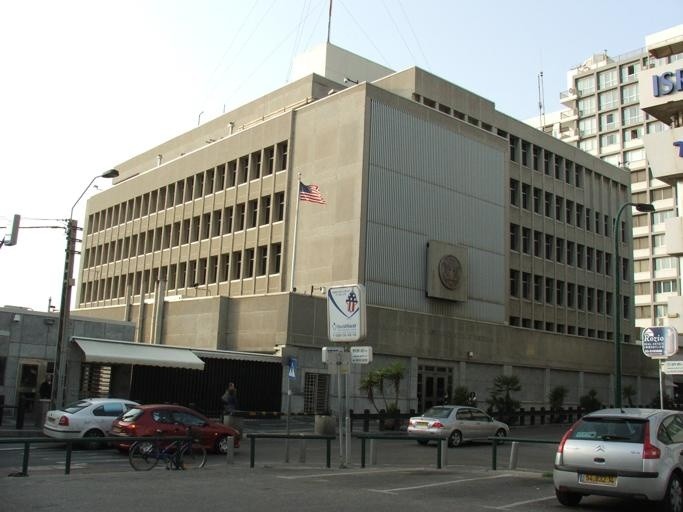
[224,415,242,441]
[314,415,336,435]
[35,399,51,429]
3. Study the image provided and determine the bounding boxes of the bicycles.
[128,424,207,470]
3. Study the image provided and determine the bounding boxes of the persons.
[222,381,238,416]
[468,392,477,406]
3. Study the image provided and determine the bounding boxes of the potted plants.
[357,358,410,433]
[485,370,523,428]
[314,405,338,439]
[222,408,245,440]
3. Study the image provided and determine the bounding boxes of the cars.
[43,396,142,438]
[108,405,242,456]
[404,403,510,447]
[550,404,682,511]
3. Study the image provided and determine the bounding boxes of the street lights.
[52,170,119,410]
[614,200,656,407]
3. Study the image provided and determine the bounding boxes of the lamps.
[13,312,22,326]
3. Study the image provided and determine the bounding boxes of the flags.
[299,181,327,204]
